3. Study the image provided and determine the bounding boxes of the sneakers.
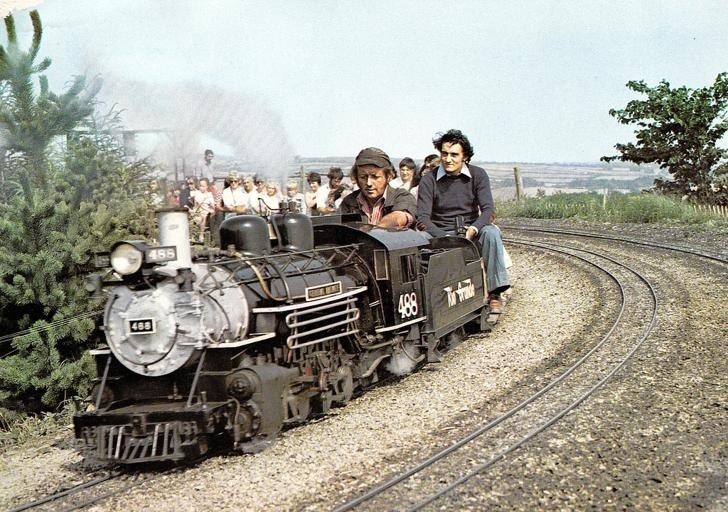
[489,299,503,314]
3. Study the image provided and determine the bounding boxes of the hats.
[353,146,392,169]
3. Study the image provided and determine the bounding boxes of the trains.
[61,206,497,475]
